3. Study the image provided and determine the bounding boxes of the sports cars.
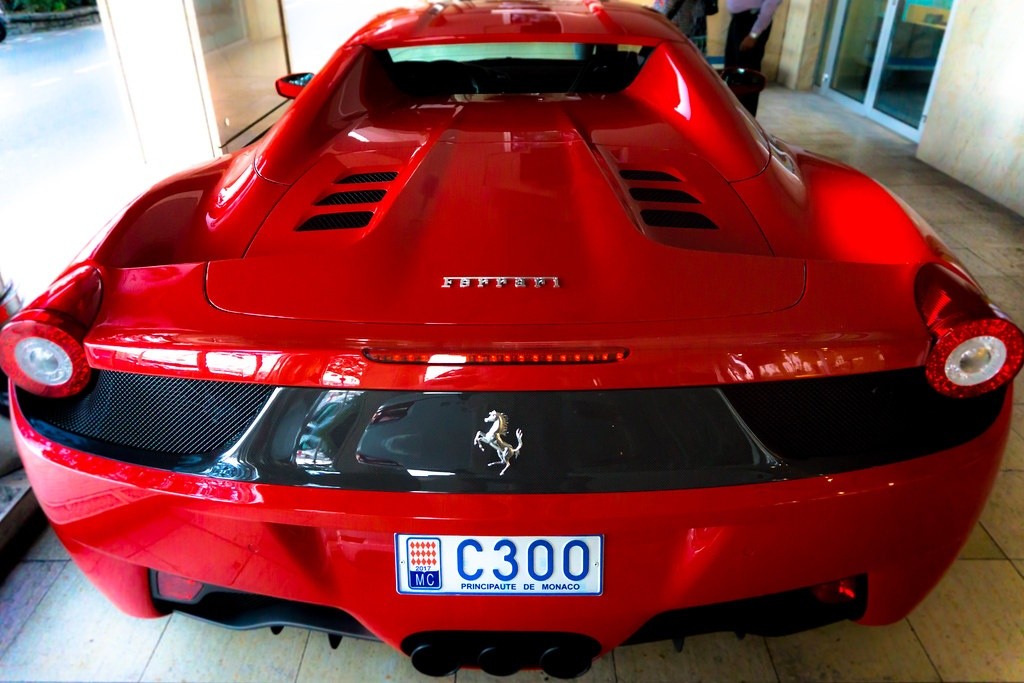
[1,0,1024,683]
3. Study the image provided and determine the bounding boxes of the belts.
[732,8,760,18]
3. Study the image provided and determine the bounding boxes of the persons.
[724,0,783,118]
[652,0,719,57]
